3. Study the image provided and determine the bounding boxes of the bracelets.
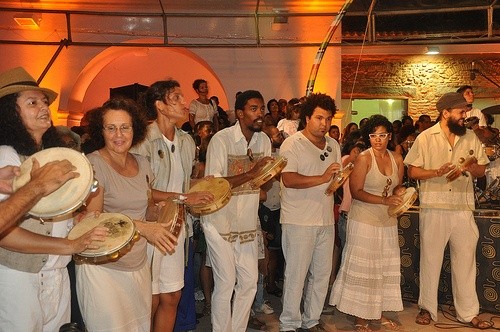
[382,196,385,203]
[137,222,147,234]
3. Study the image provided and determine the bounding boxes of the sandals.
[468,317,490,329]
[415,308,431,325]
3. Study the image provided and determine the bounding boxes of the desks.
[399,207,500,315]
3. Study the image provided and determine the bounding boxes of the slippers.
[371,318,400,330]
[355,321,372,332]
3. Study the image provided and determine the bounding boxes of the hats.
[0,67,58,106]
[436,92,472,112]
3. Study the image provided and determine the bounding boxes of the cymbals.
[473,126,499,144]
[248,154,289,190]
[324,162,355,197]
[464,116,480,130]
[155,196,186,246]
[67,212,141,267]
[480,105,500,115]
[184,176,233,217]
[10,147,100,225]
[445,156,479,182]
[387,186,419,218]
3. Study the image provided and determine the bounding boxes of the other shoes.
[202,303,211,316]
[300,324,329,332]
[248,315,266,330]
[267,287,282,296]
[255,303,274,314]
[195,290,205,303]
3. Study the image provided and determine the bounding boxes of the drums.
[389,187,418,217]
[248,155,288,191]
[446,157,478,181]
[11,147,94,222]
[482,145,498,161]
[156,201,184,245]
[324,163,353,197]
[185,178,233,217]
[464,117,479,129]
[68,213,140,265]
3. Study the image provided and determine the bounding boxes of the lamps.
[271,14,289,32]
[423,46,440,54]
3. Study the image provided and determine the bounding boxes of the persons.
[1,79,500,332]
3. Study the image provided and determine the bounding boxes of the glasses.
[369,133,389,140]
[100,124,133,134]
